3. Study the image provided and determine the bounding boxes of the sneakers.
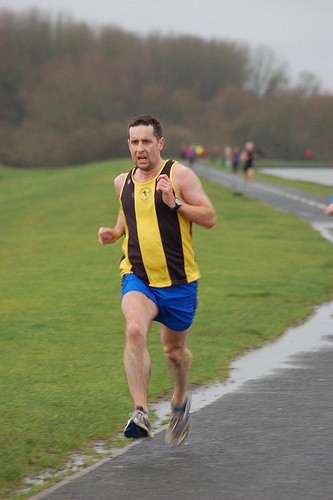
[165,391,191,448]
[123,406,154,441]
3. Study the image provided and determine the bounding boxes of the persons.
[98,114,217,447]
[240,141,259,186]
[180,142,195,166]
[230,146,241,173]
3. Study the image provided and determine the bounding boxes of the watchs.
[170,197,183,212]
